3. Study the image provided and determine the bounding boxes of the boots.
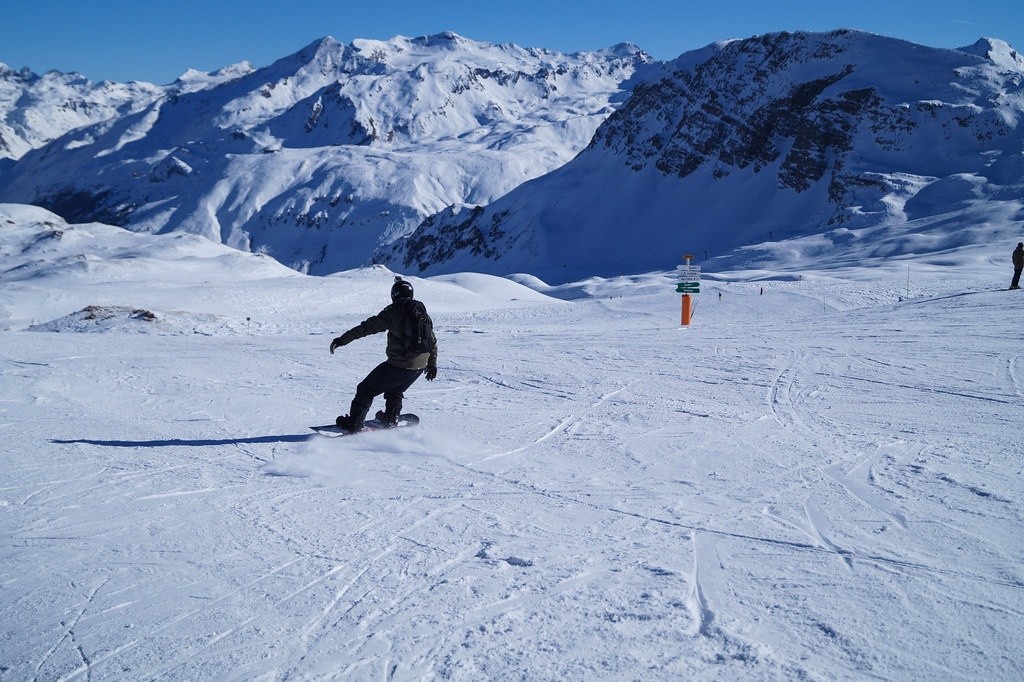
[336,407,368,431]
[375,409,398,428]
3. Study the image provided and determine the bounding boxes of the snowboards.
[308,413,420,438]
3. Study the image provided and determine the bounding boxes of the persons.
[329,280,437,433]
[1009,242,1024,289]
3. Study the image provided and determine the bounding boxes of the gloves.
[329,338,342,354]
[423,366,437,381]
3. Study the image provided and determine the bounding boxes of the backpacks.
[400,299,433,355]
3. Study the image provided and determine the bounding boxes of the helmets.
[390,281,414,299]
